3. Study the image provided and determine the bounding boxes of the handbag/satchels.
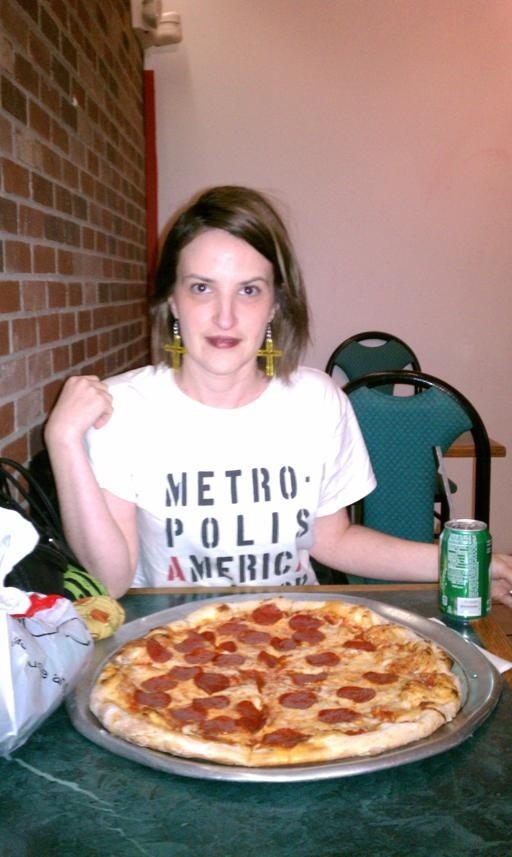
[0,455,68,596]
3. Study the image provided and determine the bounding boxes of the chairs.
[324,331,458,543]
[334,372,493,571]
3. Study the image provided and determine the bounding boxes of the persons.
[38,182,511,612]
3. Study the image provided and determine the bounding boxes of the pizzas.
[88,599,462,767]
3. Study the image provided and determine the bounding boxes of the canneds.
[438,518,492,623]
